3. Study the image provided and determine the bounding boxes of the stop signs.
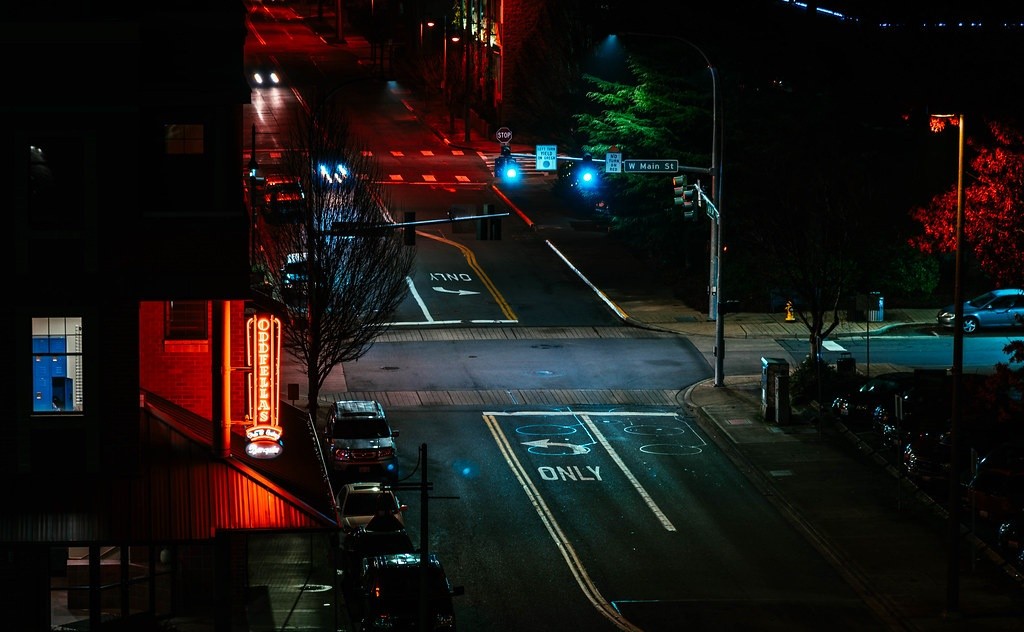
[496,127,512,144]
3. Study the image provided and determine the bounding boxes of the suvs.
[323,400,400,486]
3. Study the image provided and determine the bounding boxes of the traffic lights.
[581,153,595,185]
[683,188,698,222]
[505,158,519,182]
[672,175,685,205]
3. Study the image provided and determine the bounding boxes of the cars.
[334,481,408,551]
[247,50,283,87]
[280,250,330,308]
[358,551,465,631]
[936,286,1024,334]
[262,174,307,221]
[312,145,350,187]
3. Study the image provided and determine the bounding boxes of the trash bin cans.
[757,355,791,425]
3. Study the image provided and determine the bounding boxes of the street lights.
[426,20,473,144]
[929,108,968,378]
[608,28,720,325]
